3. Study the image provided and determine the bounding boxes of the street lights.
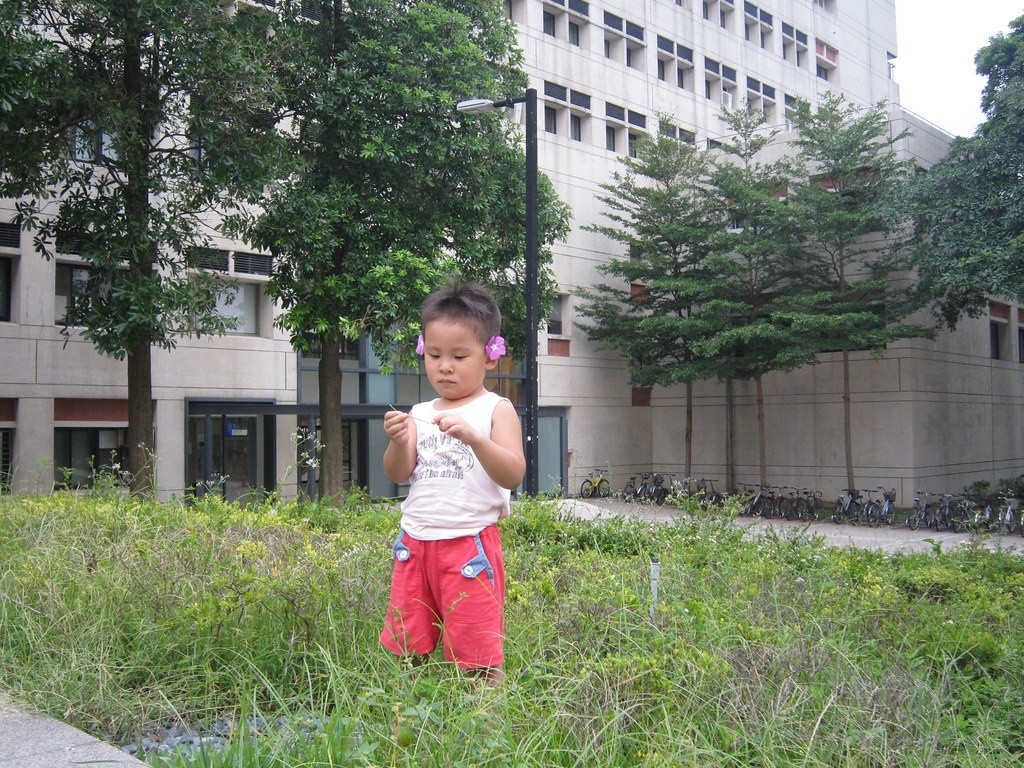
[456,88,539,500]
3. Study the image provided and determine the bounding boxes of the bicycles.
[623,471,825,522]
[832,485,898,527]
[906,489,1024,537]
[581,468,610,499]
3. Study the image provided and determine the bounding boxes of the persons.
[382,278,527,690]
